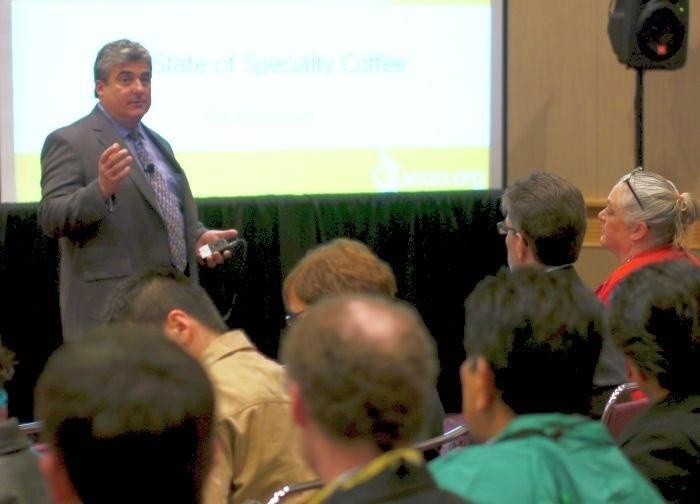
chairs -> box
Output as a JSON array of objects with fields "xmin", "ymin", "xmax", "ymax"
[
  {"xmin": 266, "ymin": 423, "xmax": 471, "ymax": 502},
  {"xmin": 602, "ymin": 381, "xmax": 655, "ymax": 437},
  {"xmin": 15, "ymin": 418, "xmax": 83, "ymax": 503}
]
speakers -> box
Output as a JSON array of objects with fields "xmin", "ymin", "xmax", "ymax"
[{"xmin": 607, "ymin": 0, "xmax": 690, "ymax": 71}]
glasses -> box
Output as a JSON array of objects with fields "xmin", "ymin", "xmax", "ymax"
[
  {"xmin": 622, "ymin": 166, "xmax": 652, "ymax": 208},
  {"xmin": 496, "ymin": 221, "xmax": 530, "ymax": 245},
  {"xmin": 284, "ymin": 308, "xmax": 307, "ymax": 326}
]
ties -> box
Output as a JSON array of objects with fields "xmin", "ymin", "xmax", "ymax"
[{"xmin": 129, "ymin": 131, "xmax": 187, "ymax": 274}]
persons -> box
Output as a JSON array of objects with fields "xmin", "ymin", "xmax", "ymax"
[
  {"xmin": 497, "ymin": 168, "xmax": 633, "ymax": 388},
  {"xmin": 592, "ymin": 166, "xmax": 700, "ymax": 402},
  {"xmin": 33, "ymin": 36, "xmax": 244, "ymax": 347},
  {"xmin": 2, "ymin": 239, "xmax": 700, "ymax": 504}
]
